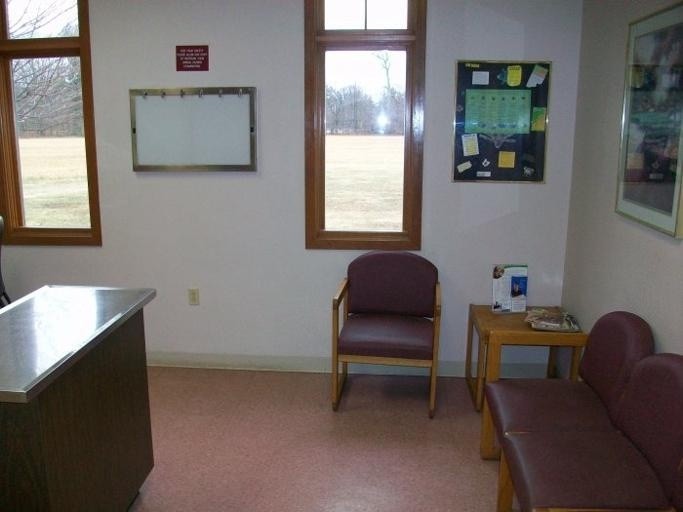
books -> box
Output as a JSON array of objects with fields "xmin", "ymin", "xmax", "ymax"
[{"xmin": 522, "ymin": 305, "xmax": 567, "ymax": 328}]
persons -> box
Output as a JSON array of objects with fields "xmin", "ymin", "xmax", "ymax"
[{"xmin": 511, "ymin": 281, "xmax": 522, "ymax": 296}]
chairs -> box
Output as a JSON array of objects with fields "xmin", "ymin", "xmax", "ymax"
[
  {"xmin": 478, "ymin": 311, "xmax": 657, "ymax": 510},
  {"xmin": 495, "ymin": 349, "xmax": 682, "ymax": 511},
  {"xmin": 326, "ymin": 250, "xmax": 443, "ymax": 419}
]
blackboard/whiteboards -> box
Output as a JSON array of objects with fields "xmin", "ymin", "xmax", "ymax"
[{"xmin": 128, "ymin": 87, "xmax": 257, "ymax": 172}]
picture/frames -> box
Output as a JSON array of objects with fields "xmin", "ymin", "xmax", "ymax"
[{"xmin": 613, "ymin": 0, "xmax": 682, "ymax": 240}]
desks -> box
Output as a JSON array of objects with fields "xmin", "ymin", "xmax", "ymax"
[{"xmin": 0, "ymin": 283, "xmax": 158, "ymax": 512}]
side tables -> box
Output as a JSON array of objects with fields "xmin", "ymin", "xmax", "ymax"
[{"xmin": 465, "ymin": 303, "xmax": 586, "ymax": 411}]
